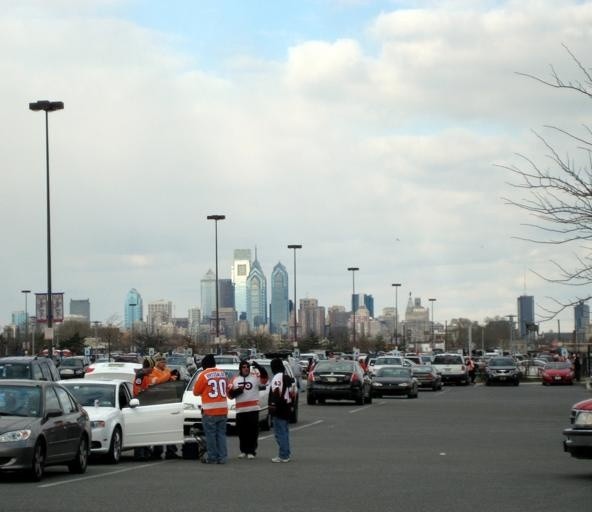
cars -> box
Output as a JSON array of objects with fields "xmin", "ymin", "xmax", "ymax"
[{"xmin": 563, "ymin": 398, "xmax": 591, "ymax": 460}]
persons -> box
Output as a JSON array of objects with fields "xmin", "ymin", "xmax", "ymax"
[
  {"xmin": 267, "ymin": 358, "xmax": 298, "ymax": 463},
  {"xmin": 193, "ymin": 354, "xmax": 230, "ymax": 465},
  {"xmin": 574, "ymin": 357, "xmax": 580, "ymax": 380},
  {"xmin": 133, "ymin": 357, "xmax": 154, "ymax": 462},
  {"xmin": 466, "ymin": 356, "xmax": 475, "ymax": 383},
  {"xmin": 227, "ymin": 361, "xmax": 269, "ymax": 459},
  {"xmin": 151, "ymin": 358, "xmax": 181, "ymax": 460}
]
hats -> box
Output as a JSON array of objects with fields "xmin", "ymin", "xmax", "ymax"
[{"xmin": 143, "ymin": 357, "xmax": 154, "ymax": 368}]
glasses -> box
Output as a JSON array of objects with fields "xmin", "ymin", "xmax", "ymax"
[{"xmin": 242, "ymin": 366, "xmax": 249, "ymax": 369}]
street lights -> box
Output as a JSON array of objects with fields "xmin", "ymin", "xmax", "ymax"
[
  {"xmin": 207, "ymin": 215, "xmax": 226, "ymax": 353},
  {"xmin": 22, "ymin": 288, "xmax": 31, "ymax": 352},
  {"xmin": 391, "ymin": 283, "xmax": 401, "ymax": 346},
  {"xmin": 503, "ymin": 313, "xmax": 518, "ymax": 352},
  {"xmin": 129, "ymin": 303, "xmax": 138, "ymax": 352},
  {"xmin": 428, "ymin": 298, "xmax": 436, "ymax": 351},
  {"xmin": 28, "ymin": 99, "xmax": 65, "ymax": 358},
  {"xmin": 287, "ymin": 244, "xmax": 302, "ymax": 357},
  {"xmin": 347, "ymin": 267, "xmax": 359, "ymax": 349},
  {"xmin": 91, "ymin": 319, "xmax": 103, "ymax": 361}
]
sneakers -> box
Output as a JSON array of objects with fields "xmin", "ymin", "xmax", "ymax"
[
  {"xmin": 133, "ymin": 454, "xmax": 182, "ymax": 462},
  {"xmin": 271, "ymin": 456, "xmax": 291, "ymax": 463},
  {"xmin": 200, "ymin": 456, "xmax": 224, "ymax": 465},
  {"xmin": 237, "ymin": 452, "xmax": 255, "ymax": 460}
]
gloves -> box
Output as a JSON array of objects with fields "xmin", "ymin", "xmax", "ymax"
[
  {"xmin": 230, "ymin": 388, "xmax": 243, "ymax": 398},
  {"xmin": 252, "ymin": 361, "xmax": 263, "ymax": 371}
]
